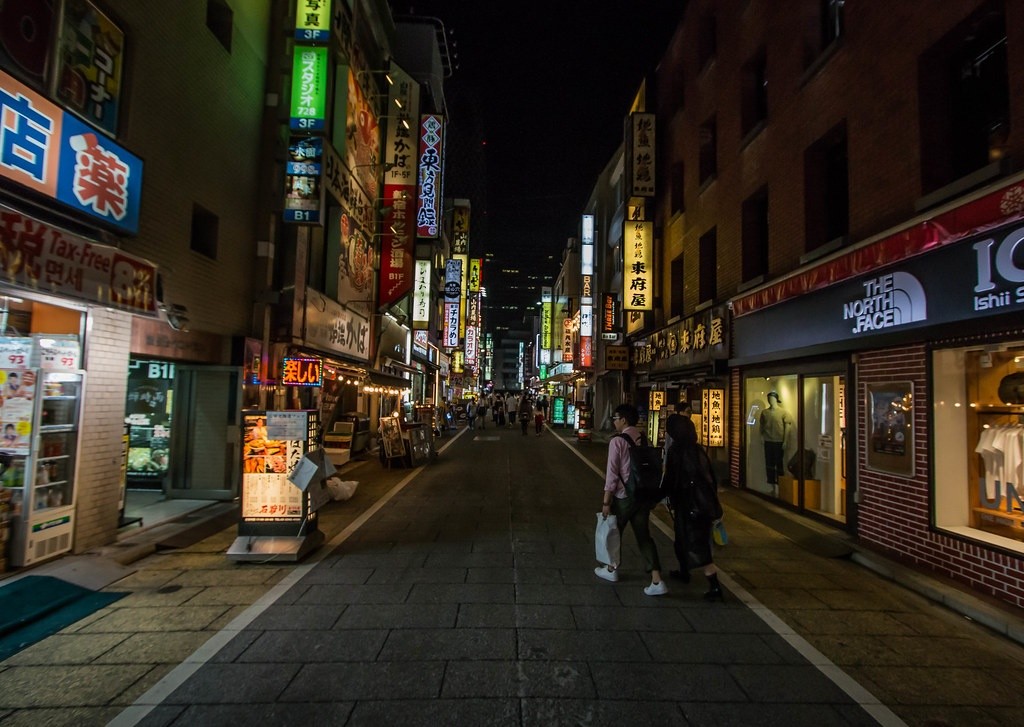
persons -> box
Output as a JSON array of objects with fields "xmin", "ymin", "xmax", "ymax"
[
  {"xmin": 3, "ymin": 374, "xmax": 26, "ymax": 401},
  {"xmin": 663, "ymin": 401, "xmax": 691, "ymax": 463},
  {"xmin": 595, "ymin": 402, "xmax": 669, "ymax": 596},
  {"xmin": 659, "ymin": 414, "xmax": 724, "ymax": 598},
  {"xmin": 0, "ymin": 422, "xmax": 18, "ymax": 449},
  {"xmin": 466, "ymin": 388, "xmax": 549, "ymax": 436},
  {"xmin": 758, "ymin": 392, "xmax": 792, "ymax": 496}
]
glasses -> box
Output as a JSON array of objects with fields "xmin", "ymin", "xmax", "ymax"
[
  {"xmin": 609, "ymin": 416, "xmax": 622, "ymax": 422},
  {"xmin": 685, "ymin": 410, "xmax": 693, "ymax": 414}
]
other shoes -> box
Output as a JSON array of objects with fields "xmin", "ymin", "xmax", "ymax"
[
  {"xmin": 536, "ymin": 432, "xmax": 542, "ymax": 436},
  {"xmin": 509, "ymin": 422, "xmax": 513, "ymax": 429}
]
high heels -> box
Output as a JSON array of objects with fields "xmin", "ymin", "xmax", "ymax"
[
  {"xmin": 670, "ymin": 568, "xmax": 694, "ymax": 593},
  {"xmin": 702, "ymin": 586, "xmax": 729, "ymax": 607}
]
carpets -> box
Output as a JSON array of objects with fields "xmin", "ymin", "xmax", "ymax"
[{"xmin": 0, "ymin": 574, "xmax": 133, "ymax": 663}]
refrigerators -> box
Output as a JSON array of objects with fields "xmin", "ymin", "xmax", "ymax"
[{"xmin": 11, "ymin": 368, "xmax": 88, "ymax": 568}]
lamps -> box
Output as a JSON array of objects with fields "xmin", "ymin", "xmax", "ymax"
[
  {"xmin": 367, "ymin": 313, "xmax": 406, "ymax": 327},
  {"xmin": 363, "ymin": 94, "xmax": 407, "ymax": 108},
  {"xmin": 375, "ymin": 116, "xmax": 415, "ymax": 129},
  {"xmin": 351, "ymin": 162, "xmax": 396, "ymax": 172},
  {"xmin": 976, "ymin": 351, "xmax": 993, "ymax": 369},
  {"xmin": 355, "ymin": 70, "xmax": 399, "ymax": 85},
  {"xmin": 343, "ymin": 300, "xmax": 389, "ymax": 314},
  {"xmin": 158, "ymin": 305, "xmax": 191, "ymax": 332},
  {"xmin": 355, "ymin": 190, "xmax": 412, "ymax": 245}
]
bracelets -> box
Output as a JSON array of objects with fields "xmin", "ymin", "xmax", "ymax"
[{"xmin": 602, "ymin": 503, "xmax": 609, "ymax": 506}]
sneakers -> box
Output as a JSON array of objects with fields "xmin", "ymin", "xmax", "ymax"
[
  {"xmin": 644, "ymin": 581, "xmax": 668, "ymax": 595},
  {"xmin": 593, "ymin": 565, "xmax": 618, "ymax": 582}
]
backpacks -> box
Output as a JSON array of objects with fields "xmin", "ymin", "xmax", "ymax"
[
  {"xmin": 788, "ymin": 447, "xmax": 817, "ymax": 480},
  {"xmin": 609, "ymin": 430, "xmax": 664, "ymax": 511},
  {"xmin": 671, "ymin": 444, "xmax": 723, "ymax": 523}
]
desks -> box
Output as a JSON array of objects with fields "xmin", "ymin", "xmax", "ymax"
[{"xmin": 778, "ymin": 476, "xmax": 822, "ymax": 510}]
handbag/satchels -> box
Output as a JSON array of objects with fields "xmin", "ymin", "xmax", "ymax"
[
  {"xmin": 478, "ymin": 406, "xmax": 486, "ymax": 417},
  {"xmin": 713, "ymin": 520, "xmax": 730, "ymax": 546},
  {"xmin": 594, "ymin": 512, "xmax": 620, "ymax": 569}
]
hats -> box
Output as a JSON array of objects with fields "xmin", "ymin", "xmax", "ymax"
[
  {"xmin": 544, "ymin": 395, "xmax": 547, "ymax": 398},
  {"xmin": 768, "ymin": 392, "xmax": 781, "ymax": 403}
]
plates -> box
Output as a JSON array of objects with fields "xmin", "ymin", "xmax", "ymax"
[{"xmin": 241, "ymin": 415, "xmax": 288, "ymax": 473}]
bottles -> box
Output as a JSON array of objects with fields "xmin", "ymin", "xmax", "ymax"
[{"xmin": 32, "ymin": 411, "xmax": 64, "ymax": 510}]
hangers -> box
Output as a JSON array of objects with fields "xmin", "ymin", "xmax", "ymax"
[{"xmin": 988, "ymin": 412, "xmax": 1024, "ymax": 429}]
